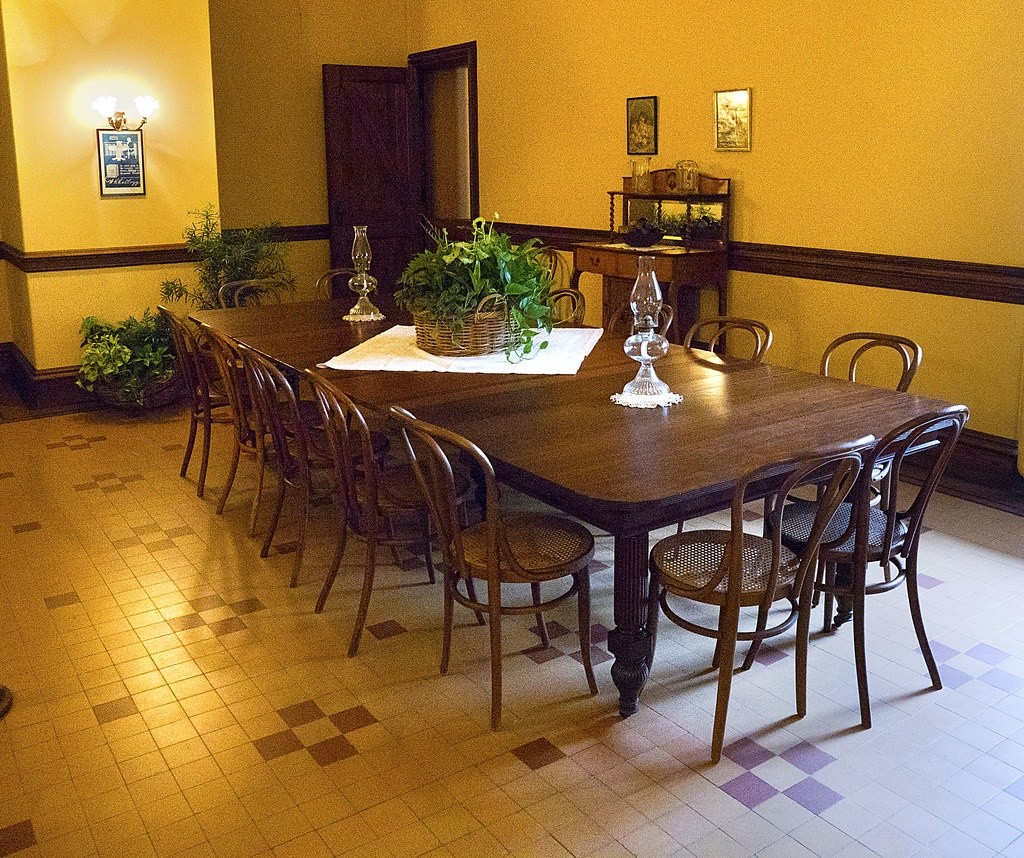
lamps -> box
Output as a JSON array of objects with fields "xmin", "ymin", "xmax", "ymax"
[{"xmin": 94, "ymin": 91, "xmax": 163, "ymax": 133}]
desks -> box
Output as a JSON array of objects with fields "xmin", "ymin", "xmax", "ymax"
[{"xmin": 186, "ymin": 301, "xmax": 972, "ymax": 721}]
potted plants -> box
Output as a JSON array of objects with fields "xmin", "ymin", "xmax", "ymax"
[
  {"xmin": 67, "ymin": 304, "xmax": 184, "ymax": 414},
  {"xmin": 392, "ymin": 211, "xmax": 564, "ymax": 368}
]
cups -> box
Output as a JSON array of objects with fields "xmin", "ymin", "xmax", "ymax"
[{"xmin": 632, "ymin": 157, "xmax": 650, "ymax": 191}]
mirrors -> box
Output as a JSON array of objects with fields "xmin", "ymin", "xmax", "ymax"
[{"xmin": 625, "ymin": 196, "xmax": 726, "ymax": 240}]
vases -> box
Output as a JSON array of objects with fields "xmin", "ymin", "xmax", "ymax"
[{"xmin": 340, "ymin": 225, "xmax": 387, "ymax": 322}]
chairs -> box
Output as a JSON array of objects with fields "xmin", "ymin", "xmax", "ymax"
[
  {"xmin": 235, "ymin": 339, "xmax": 411, "ymax": 587},
  {"xmin": 740, "ymin": 402, "xmax": 970, "ymax": 728},
  {"xmin": 299, "ymin": 367, "xmax": 488, "ymax": 659},
  {"xmin": 200, "ymin": 322, "xmax": 386, "ymax": 531},
  {"xmin": 607, "ymin": 297, "xmax": 674, "ymax": 339},
  {"xmin": 388, "ymin": 400, "xmax": 602, "ymax": 733},
  {"xmin": 148, "ymin": 303, "xmax": 332, "ymax": 497},
  {"xmin": 632, "ymin": 447, "xmax": 865, "ymax": 765},
  {"xmin": 314, "ymin": 267, "xmax": 379, "ymax": 301},
  {"xmin": 681, "ymin": 312, "xmax": 776, "ymax": 362},
  {"xmin": 547, "ymin": 283, "xmax": 587, "ymax": 327},
  {"xmin": 818, "ymin": 331, "xmax": 924, "ymax": 391},
  {"xmin": 217, "ymin": 276, "xmax": 295, "ymax": 309}
]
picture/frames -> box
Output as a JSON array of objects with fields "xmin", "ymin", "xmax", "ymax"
[
  {"xmin": 99, "ymin": 128, "xmax": 146, "ymax": 199},
  {"xmin": 624, "ymin": 94, "xmax": 660, "ymax": 156},
  {"xmin": 711, "ymin": 85, "xmax": 753, "ymax": 154}
]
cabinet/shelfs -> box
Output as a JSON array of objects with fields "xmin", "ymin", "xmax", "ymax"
[{"xmin": 568, "ymin": 167, "xmax": 732, "ymax": 355}]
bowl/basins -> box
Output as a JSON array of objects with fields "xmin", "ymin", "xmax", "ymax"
[{"xmin": 614, "ymin": 228, "xmax": 666, "ymax": 246}]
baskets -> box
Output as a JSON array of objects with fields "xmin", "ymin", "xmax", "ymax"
[{"xmin": 407, "ymin": 293, "xmax": 522, "ymax": 357}]
